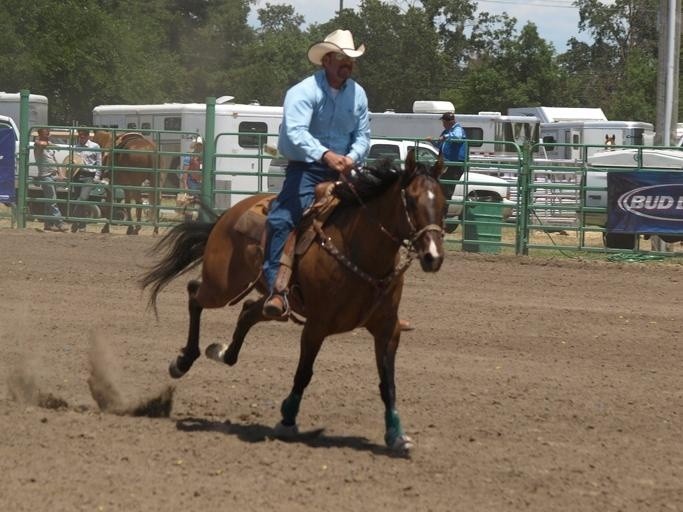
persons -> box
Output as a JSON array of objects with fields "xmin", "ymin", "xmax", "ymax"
[
  {"xmin": 33, "ymin": 128, "xmax": 68, "ymax": 232},
  {"xmin": 259, "ymin": 27, "xmax": 416, "ymax": 332},
  {"xmin": 70, "ymin": 124, "xmax": 103, "ymax": 233},
  {"xmin": 179, "ymin": 136, "xmax": 203, "ymax": 222},
  {"xmin": 422, "ymin": 111, "xmax": 469, "ymax": 219}
]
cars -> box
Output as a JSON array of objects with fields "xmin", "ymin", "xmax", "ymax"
[{"xmin": 25, "ymin": 126, "xmax": 97, "ymax": 143}]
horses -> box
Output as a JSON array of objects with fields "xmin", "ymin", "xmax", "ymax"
[
  {"xmin": 92, "ymin": 129, "xmax": 161, "ymax": 236},
  {"xmin": 604, "ymin": 133, "xmax": 617, "ymax": 152},
  {"xmin": 135, "ymin": 143, "xmax": 450, "ymax": 457}
]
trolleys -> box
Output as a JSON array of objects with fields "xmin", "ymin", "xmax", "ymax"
[{"xmin": 84, "ymin": 183, "xmax": 129, "ymax": 227}]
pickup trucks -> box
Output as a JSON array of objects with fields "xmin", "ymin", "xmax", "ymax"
[
  {"xmin": 0, "ymin": 115, "xmax": 94, "ymax": 215},
  {"xmin": 575, "ymin": 136, "xmax": 682, "ymax": 250},
  {"xmin": 267, "ymin": 136, "xmax": 514, "ymax": 234}
]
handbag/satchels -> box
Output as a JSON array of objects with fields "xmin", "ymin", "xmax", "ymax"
[{"xmin": 175, "ymin": 193, "xmax": 195, "ymax": 216}]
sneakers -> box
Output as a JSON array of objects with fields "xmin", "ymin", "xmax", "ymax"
[
  {"xmin": 59, "ymin": 222, "xmax": 69, "ymax": 231},
  {"xmin": 44, "ymin": 223, "xmax": 60, "ymax": 232}
]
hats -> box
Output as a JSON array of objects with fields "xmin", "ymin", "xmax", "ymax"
[
  {"xmin": 189, "ymin": 136, "xmax": 202, "ymax": 148},
  {"xmin": 439, "ymin": 112, "xmax": 455, "ymax": 121},
  {"xmin": 307, "ymin": 28, "xmax": 366, "ymax": 66},
  {"xmin": 61, "ymin": 155, "xmax": 84, "ymax": 179}
]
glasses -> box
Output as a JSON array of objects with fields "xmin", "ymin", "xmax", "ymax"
[{"xmin": 336, "ymin": 52, "xmax": 357, "ymax": 62}]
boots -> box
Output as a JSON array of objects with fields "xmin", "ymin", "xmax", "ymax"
[
  {"xmin": 397, "ymin": 316, "xmax": 415, "ymax": 332},
  {"xmin": 265, "ymin": 231, "xmax": 297, "ymax": 323}
]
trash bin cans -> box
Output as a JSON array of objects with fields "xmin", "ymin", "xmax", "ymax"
[{"xmin": 463, "ymin": 200, "xmax": 504, "ymax": 252}]
